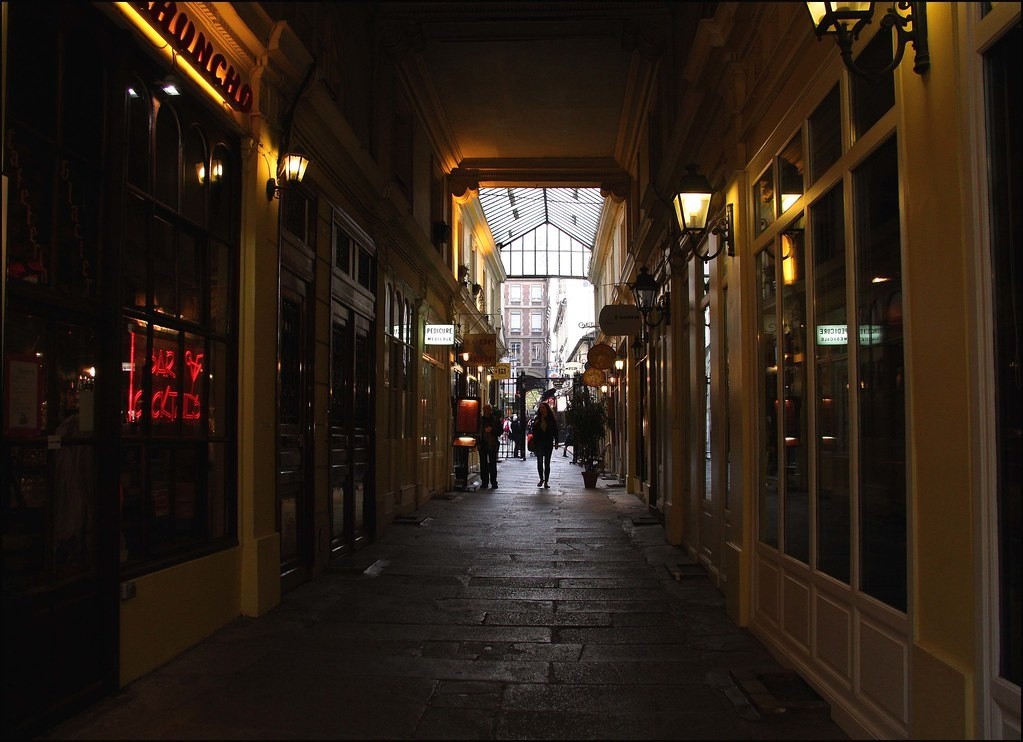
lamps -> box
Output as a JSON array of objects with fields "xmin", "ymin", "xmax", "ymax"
[
  {"xmin": 196, "ymin": 150, "xmax": 223, "ymax": 195},
  {"xmin": 629, "ymin": 267, "xmax": 670, "ymax": 328},
  {"xmin": 669, "ymin": 162, "xmax": 735, "ymax": 264},
  {"xmin": 162, "ymin": 47, "xmax": 183, "ymax": 95},
  {"xmin": 805, "ymin": 1, "xmax": 930, "ymax": 77},
  {"xmin": 631, "ymin": 335, "xmax": 647, "ymax": 362},
  {"xmin": 760, "ymin": 159, "xmax": 805, "ymax": 260},
  {"xmin": 267, "ymin": 150, "xmax": 310, "ymax": 201}
]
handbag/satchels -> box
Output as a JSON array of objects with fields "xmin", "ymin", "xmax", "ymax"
[{"xmin": 528, "ymin": 437, "xmax": 536, "ymax": 451}]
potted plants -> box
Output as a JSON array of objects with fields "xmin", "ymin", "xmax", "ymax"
[
  {"xmin": 565, "ymin": 387, "xmax": 616, "ymax": 488},
  {"xmin": 459, "ymin": 261, "xmax": 471, "ymax": 278},
  {"xmin": 472, "ymin": 283, "xmax": 485, "ymax": 317}
]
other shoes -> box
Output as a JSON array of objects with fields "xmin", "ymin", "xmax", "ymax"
[
  {"xmin": 480, "ymin": 482, "xmax": 488, "ymax": 488},
  {"xmin": 537, "ymin": 480, "xmax": 544, "ymax": 486},
  {"xmin": 492, "ymin": 484, "xmax": 498, "ymax": 489},
  {"xmin": 563, "ymin": 454, "xmax": 568, "ymax": 457},
  {"xmin": 544, "ymin": 484, "xmax": 550, "ymax": 488}
]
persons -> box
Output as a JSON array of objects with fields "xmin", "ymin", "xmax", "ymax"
[
  {"xmin": 476, "ymin": 403, "xmax": 504, "ymax": 489},
  {"xmin": 42, "ymin": 348, "xmax": 127, "ymax": 557},
  {"xmin": 509, "ymin": 403, "xmax": 573, "ymax": 488}
]
